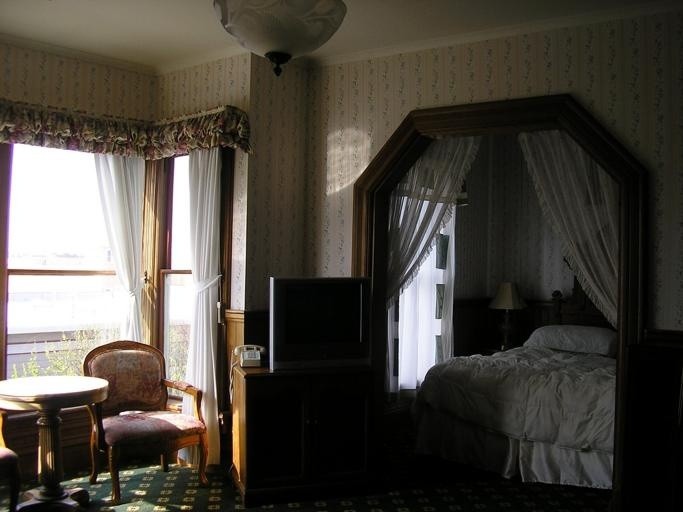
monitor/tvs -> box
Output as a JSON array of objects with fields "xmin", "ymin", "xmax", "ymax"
[{"xmin": 268, "ymin": 276, "xmax": 372, "ymax": 372}]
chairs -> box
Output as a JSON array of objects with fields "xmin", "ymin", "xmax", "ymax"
[{"xmin": 82, "ymin": 338, "xmax": 211, "ymax": 504}]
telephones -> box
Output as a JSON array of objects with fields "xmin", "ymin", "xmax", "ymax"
[{"xmin": 234, "ymin": 345, "xmax": 266, "ymax": 367}]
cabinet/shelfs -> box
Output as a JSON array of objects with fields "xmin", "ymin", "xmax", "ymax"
[{"xmin": 227, "ymin": 360, "xmax": 390, "ymax": 509}]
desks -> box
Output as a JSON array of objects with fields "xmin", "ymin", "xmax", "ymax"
[{"xmin": 0, "ymin": 374, "xmax": 110, "ymax": 508}]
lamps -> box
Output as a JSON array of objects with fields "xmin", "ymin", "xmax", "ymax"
[
  {"xmin": 489, "ymin": 281, "xmax": 525, "ymax": 347},
  {"xmin": 211, "ymin": 1, "xmax": 349, "ymax": 76}
]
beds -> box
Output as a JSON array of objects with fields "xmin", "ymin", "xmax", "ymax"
[{"xmin": 422, "ymin": 288, "xmax": 616, "ymax": 493}]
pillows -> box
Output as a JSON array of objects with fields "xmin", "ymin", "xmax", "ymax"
[{"xmin": 522, "ymin": 322, "xmax": 614, "ymax": 357}]
